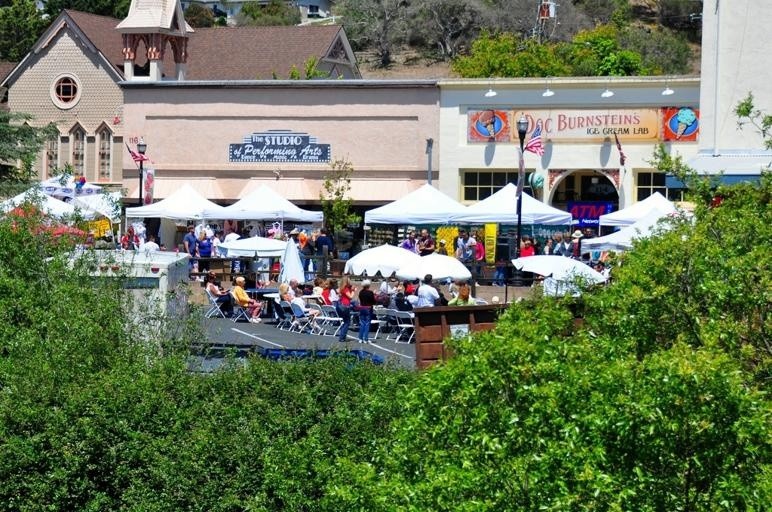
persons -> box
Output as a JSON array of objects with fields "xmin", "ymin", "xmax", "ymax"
[
  {"xmin": 492, "ymin": 246, "xmax": 505, "ymax": 287},
  {"xmin": 229, "ymin": 276, "xmax": 263, "ymax": 323},
  {"xmin": 183, "ymin": 224, "xmax": 240, "ymax": 282},
  {"xmin": 203, "ymin": 271, "xmax": 233, "ymax": 318},
  {"xmin": 399, "ymin": 229, "xmax": 485, "ymax": 287},
  {"xmin": 336, "ymin": 275, "xmax": 374, "ymax": 344},
  {"xmin": 519, "ymin": 227, "xmax": 595, "ymax": 264},
  {"xmin": 380, "ymin": 274, "xmax": 476, "ymax": 343},
  {"xmin": 250, "ymin": 272, "xmax": 340, "ymax": 334},
  {"xmin": 122, "ymin": 226, "xmax": 168, "ymax": 252},
  {"xmin": 268, "ymin": 226, "xmax": 332, "ymax": 279}
]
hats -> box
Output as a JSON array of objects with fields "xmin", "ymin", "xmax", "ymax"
[
  {"xmin": 572, "ymin": 230, "xmax": 583, "ymax": 238},
  {"xmin": 288, "ymin": 231, "xmax": 299, "ymax": 238},
  {"xmin": 268, "ymin": 229, "xmax": 277, "ymax": 234},
  {"xmin": 405, "ymin": 284, "xmax": 417, "ymax": 294},
  {"xmin": 438, "ymin": 239, "xmax": 446, "ymax": 245}
]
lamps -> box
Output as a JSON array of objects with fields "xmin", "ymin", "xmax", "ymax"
[
  {"xmin": 591, "ymin": 170, "xmax": 599, "ymax": 186},
  {"xmin": 601, "ymin": 89, "xmax": 613, "ymax": 99},
  {"xmin": 485, "ymin": 89, "xmax": 496, "ymax": 98},
  {"xmin": 542, "ymin": 89, "xmax": 554, "ymax": 96},
  {"xmin": 661, "ymin": 86, "xmax": 674, "ymax": 96}
]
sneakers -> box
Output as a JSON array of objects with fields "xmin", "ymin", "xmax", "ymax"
[
  {"xmin": 339, "ymin": 338, "xmax": 350, "ymax": 342},
  {"xmin": 248, "ymin": 317, "xmax": 262, "ymax": 324},
  {"xmin": 359, "ymin": 339, "xmax": 372, "ymax": 344}
]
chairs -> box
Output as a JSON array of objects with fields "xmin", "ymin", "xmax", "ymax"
[{"xmin": 202, "ymin": 287, "xmax": 414, "ymax": 346}]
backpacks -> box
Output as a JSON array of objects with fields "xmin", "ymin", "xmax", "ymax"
[{"xmin": 397, "ymin": 295, "xmax": 413, "ymax": 311}]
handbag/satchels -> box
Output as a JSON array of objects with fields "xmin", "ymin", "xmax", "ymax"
[{"xmin": 336, "ymin": 300, "xmax": 350, "ymax": 318}]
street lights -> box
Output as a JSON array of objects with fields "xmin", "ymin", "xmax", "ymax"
[
  {"xmin": 134, "ymin": 138, "xmax": 147, "ymax": 203},
  {"xmin": 503, "ymin": 113, "xmax": 529, "ymax": 286}
]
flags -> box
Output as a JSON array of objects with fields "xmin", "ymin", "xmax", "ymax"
[
  {"xmin": 525, "ymin": 123, "xmax": 544, "ymax": 157},
  {"xmin": 127, "ymin": 142, "xmax": 151, "ymax": 161}
]
